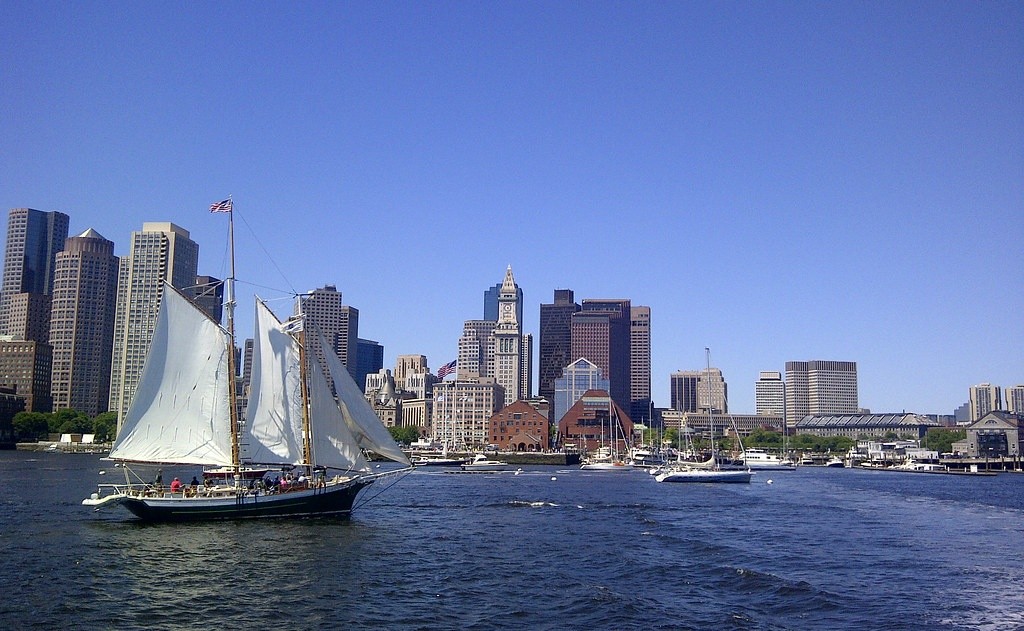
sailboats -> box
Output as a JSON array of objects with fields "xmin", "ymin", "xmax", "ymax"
[{"xmin": 80, "ymin": 195, "xmax": 418, "ymax": 522}]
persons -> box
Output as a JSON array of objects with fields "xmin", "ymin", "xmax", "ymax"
[
  {"xmin": 189, "ymin": 477, "xmax": 199, "ymax": 486},
  {"xmin": 169, "ymin": 477, "xmax": 183, "ymax": 493},
  {"xmin": 202, "ymin": 477, "xmax": 212, "ymax": 488},
  {"xmin": 255, "ymin": 473, "xmax": 323, "ymax": 495},
  {"xmin": 154, "ymin": 470, "xmax": 163, "ymax": 496}
]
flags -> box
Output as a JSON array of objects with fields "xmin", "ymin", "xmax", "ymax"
[
  {"xmin": 209, "ymin": 199, "xmax": 231, "ymax": 214},
  {"xmin": 436, "ymin": 361, "xmax": 455, "ymax": 379}
]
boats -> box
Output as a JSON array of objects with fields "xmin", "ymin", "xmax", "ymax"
[
  {"xmin": 372, "ymin": 345, "xmax": 1024, "ymax": 484},
  {"xmin": 42, "ymin": 438, "xmax": 114, "ymax": 455}
]
